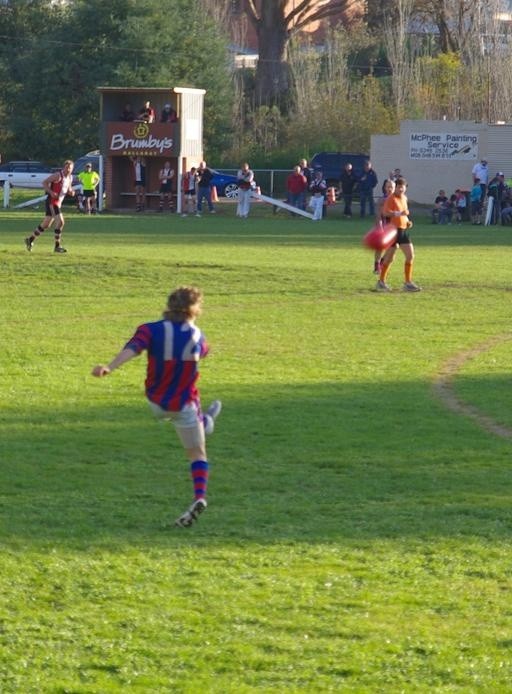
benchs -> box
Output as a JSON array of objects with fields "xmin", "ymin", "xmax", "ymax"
[{"xmin": 410, "ymin": 208, "xmax": 462, "ymax": 225}]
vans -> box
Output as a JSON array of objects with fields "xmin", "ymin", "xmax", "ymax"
[{"xmin": 65, "ymin": 150, "xmax": 101, "ymax": 201}]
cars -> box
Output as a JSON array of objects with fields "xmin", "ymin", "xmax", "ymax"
[
  {"xmin": 1, "ymin": 160, "xmax": 59, "ymax": 187},
  {"xmin": 205, "ymin": 166, "xmax": 256, "ymax": 199}
]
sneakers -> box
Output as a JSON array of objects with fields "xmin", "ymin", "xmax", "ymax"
[
  {"xmin": 24, "ymin": 237, "xmax": 34, "ymax": 253},
  {"xmin": 54, "ymin": 247, "xmax": 67, "ymax": 254},
  {"xmin": 204, "ymin": 400, "xmax": 221, "ymax": 434},
  {"xmin": 172, "ymin": 497, "xmax": 207, "ymax": 529},
  {"xmin": 375, "ymin": 280, "xmax": 393, "ymax": 292},
  {"xmin": 403, "ymin": 282, "xmax": 421, "ymax": 293}
]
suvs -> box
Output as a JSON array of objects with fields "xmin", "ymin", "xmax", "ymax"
[{"xmin": 303, "ymin": 150, "xmax": 370, "ymax": 200}]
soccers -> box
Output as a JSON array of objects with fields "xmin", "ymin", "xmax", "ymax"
[{"xmin": 367, "ymin": 222, "xmax": 400, "ymax": 248}]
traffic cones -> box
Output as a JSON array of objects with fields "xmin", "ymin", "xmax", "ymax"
[
  {"xmin": 209, "ymin": 184, "xmax": 220, "ymax": 202},
  {"xmin": 325, "ymin": 184, "xmax": 337, "ymax": 205},
  {"xmin": 253, "ymin": 186, "xmax": 263, "ymax": 202}
]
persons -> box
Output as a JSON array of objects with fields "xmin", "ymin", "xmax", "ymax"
[
  {"xmin": 24, "ymin": 159, "xmax": 75, "ymax": 253},
  {"xmin": 196, "ymin": 161, "xmax": 215, "ymax": 214},
  {"xmin": 180, "ymin": 166, "xmax": 200, "ymax": 216},
  {"xmin": 137, "ymin": 100, "xmax": 155, "ymax": 124},
  {"xmin": 234, "ymin": 163, "xmax": 254, "ymax": 220},
  {"xmin": 372, "ymin": 179, "xmax": 396, "ymax": 274},
  {"xmin": 130, "ymin": 156, "xmax": 147, "ymax": 212},
  {"xmin": 287, "ymin": 156, "xmax": 404, "ymax": 219},
  {"xmin": 120, "ymin": 102, "xmax": 138, "ymax": 121},
  {"xmin": 430, "ymin": 156, "xmax": 511, "ymax": 226},
  {"xmin": 90, "ymin": 285, "xmax": 222, "ymax": 526},
  {"xmin": 157, "ymin": 160, "xmax": 175, "ymax": 212},
  {"xmin": 78, "ymin": 162, "xmax": 100, "ymax": 215},
  {"xmin": 376, "ymin": 176, "xmax": 422, "ymax": 291},
  {"xmin": 159, "ymin": 103, "xmax": 176, "ymax": 122}
]
